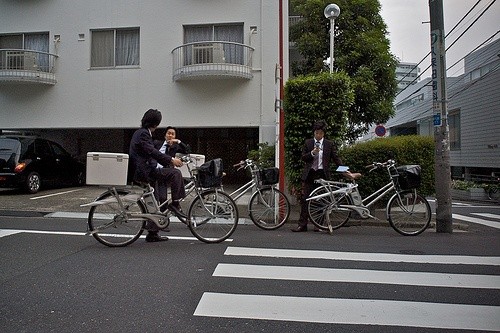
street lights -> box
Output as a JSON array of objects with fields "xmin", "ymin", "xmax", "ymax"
[{"xmin": 324, "ymin": 3, "xmax": 341, "ymax": 74}]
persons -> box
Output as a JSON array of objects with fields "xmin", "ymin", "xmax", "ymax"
[
  {"xmin": 153, "ymin": 127, "xmax": 189, "ymax": 232},
  {"xmin": 127, "ymin": 110, "xmax": 187, "ymax": 242},
  {"xmin": 290, "ymin": 122, "xmax": 351, "ymax": 232}
]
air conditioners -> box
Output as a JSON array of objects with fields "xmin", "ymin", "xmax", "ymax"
[
  {"xmin": 5, "ymin": 50, "xmax": 38, "ymax": 71},
  {"xmin": 191, "ymin": 44, "xmax": 224, "ymax": 63}
]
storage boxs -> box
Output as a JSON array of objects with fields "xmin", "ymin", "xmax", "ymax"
[
  {"xmin": 174, "ymin": 154, "xmax": 205, "ymax": 177},
  {"xmin": 85, "ymin": 151, "xmax": 129, "ymax": 187}
]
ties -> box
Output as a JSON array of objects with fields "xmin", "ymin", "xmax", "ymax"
[
  {"xmin": 165, "ymin": 144, "xmax": 168, "ymax": 155},
  {"xmin": 313, "ymin": 142, "xmax": 318, "ymax": 171}
]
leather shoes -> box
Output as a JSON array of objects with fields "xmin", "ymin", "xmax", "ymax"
[
  {"xmin": 314, "ymin": 226, "xmax": 324, "ymax": 231},
  {"xmin": 167, "ymin": 204, "xmax": 188, "ymax": 218},
  {"xmin": 146, "ymin": 235, "xmax": 167, "ymax": 241},
  {"xmin": 290, "ymin": 226, "xmax": 307, "ymax": 232}
]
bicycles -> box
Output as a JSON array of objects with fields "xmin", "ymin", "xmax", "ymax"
[
  {"xmin": 487, "ymin": 178, "xmax": 500, "ymax": 200},
  {"xmin": 305, "ymin": 159, "xmax": 432, "ymax": 236},
  {"xmin": 171, "ymin": 159, "xmax": 290, "ymax": 230},
  {"xmin": 85, "ymin": 155, "xmax": 240, "ymax": 247}
]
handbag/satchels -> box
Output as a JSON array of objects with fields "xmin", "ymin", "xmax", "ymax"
[
  {"xmin": 397, "ymin": 166, "xmax": 421, "ymax": 188},
  {"xmin": 198, "ymin": 158, "xmax": 222, "ymax": 187},
  {"xmin": 260, "ymin": 168, "xmax": 279, "ymax": 185}
]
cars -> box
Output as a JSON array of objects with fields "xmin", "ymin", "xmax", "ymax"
[{"xmin": 0, "ymin": 135, "xmax": 86, "ymax": 194}]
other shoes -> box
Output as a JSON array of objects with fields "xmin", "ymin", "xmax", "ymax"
[{"xmin": 162, "ymin": 228, "xmax": 169, "ymax": 231}]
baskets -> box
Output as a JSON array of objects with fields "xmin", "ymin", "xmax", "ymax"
[
  {"xmin": 258, "ymin": 168, "xmax": 279, "ymax": 186},
  {"xmin": 192, "ymin": 157, "xmax": 222, "ymax": 187},
  {"xmin": 393, "ymin": 165, "xmax": 421, "ymax": 189}
]
